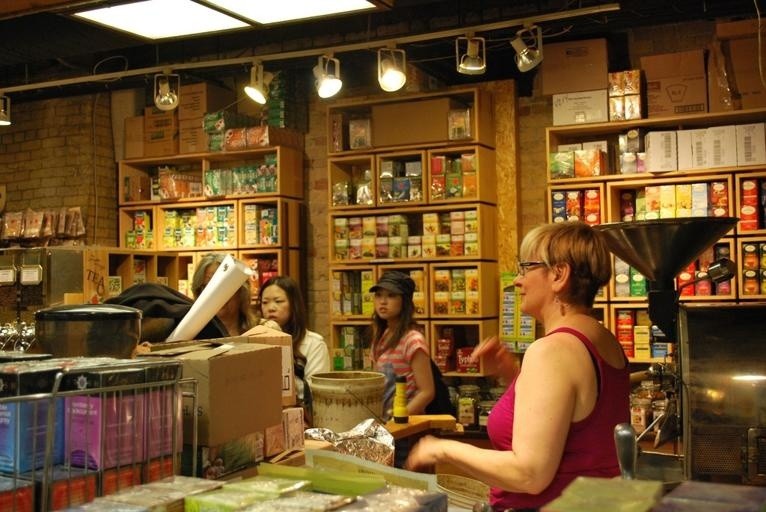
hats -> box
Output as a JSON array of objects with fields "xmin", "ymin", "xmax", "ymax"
[{"xmin": 369, "ymin": 270, "xmax": 415, "ymax": 295}]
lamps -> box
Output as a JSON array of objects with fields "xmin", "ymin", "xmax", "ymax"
[{"xmin": 0, "ymin": 0, "xmax": 623, "ymax": 126}]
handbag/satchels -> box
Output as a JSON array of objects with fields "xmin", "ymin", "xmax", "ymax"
[{"xmin": 424, "ymin": 357, "xmax": 456, "ymax": 417}]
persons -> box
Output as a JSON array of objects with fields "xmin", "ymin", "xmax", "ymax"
[
  {"xmin": 367, "ymin": 270, "xmax": 458, "ymax": 416},
  {"xmin": 191, "ymin": 253, "xmax": 284, "ymax": 337},
  {"xmin": 257, "ymin": 275, "xmax": 331, "ymax": 429},
  {"xmin": 402, "ymin": 220, "xmax": 635, "ymax": 512}
]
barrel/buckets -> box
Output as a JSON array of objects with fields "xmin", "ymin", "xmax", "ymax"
[{"xmin": 309, "ymin": 371, "xmax": 390, "ymax": 438}]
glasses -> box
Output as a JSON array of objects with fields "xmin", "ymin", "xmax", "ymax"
[{"xmin": 517, "ymin": 261, "xmax": 545, "ymax": 275}]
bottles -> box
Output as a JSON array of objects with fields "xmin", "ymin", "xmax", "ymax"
[
  {"xmin": 392, "ymin": 377, "xmax": 412, "ymax": 424},
  {"xmin": 455, "ymin": 383, "xmax": 506, "ymax": 438},
  {"xmin": 633, "ymin": 379, "xmax": 673, "ymax": 436}
]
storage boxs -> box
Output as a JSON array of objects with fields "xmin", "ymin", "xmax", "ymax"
[
  {"xmin": 370, "ymin": 97, "xmax": 450, "ymax": 146},
  {"xmin": 135, "ymin": 325, "xmax": 296, "ymax": 447},
  {"xmin": 540, "ymin": 18, "xmax": 766, "ymax": 126},
  {"xmin": 126, "ymin": 77, "xmax": 301, "ymax": 156}
]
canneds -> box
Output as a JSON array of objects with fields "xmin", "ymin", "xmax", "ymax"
[{"xmin": 448, "ymin": 384, "xmax": 508, "ymax": 434}]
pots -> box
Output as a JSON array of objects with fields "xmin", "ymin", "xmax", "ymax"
[{"xmin": 30, "ymin": 299, "xmax": 146, "ymax": 359}]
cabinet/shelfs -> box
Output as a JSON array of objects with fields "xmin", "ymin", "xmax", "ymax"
[
  {"xmin": 323, "ymin": 87, "xmax": 498, "ymax": 378},
  {"xmin": 544, "ymin": 109, "xmax": 766, "ymax": 458},
  {"xmin": 85, "ymin": 149, "xmax": 305, "ymax": 307}
]
